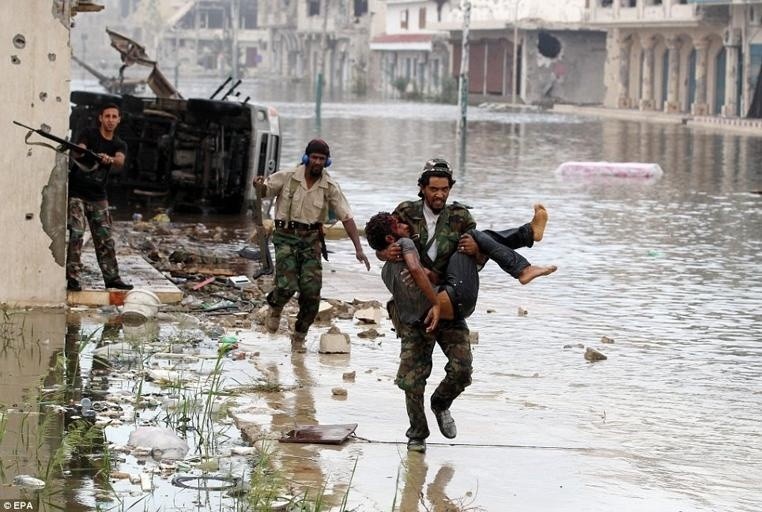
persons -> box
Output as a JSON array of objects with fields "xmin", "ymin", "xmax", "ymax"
[
  {"xmin": 252, "ymin": 139, "xmax": 371, "ymax": 352},
  {"xmin": 375, "ymin": 158, "xmax": 490, "ymax": 453},
  {"xmin": 366, "ymin": 202, "xmax": 558, "ymax": 333},
  {"xmin": 64, "ymin": 103, "xmax": 134, "ymax": 292}
]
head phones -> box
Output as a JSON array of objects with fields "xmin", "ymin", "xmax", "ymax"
[{"xmin": 302, "ymin": 138, "xmax": 332, "ymax": 169}]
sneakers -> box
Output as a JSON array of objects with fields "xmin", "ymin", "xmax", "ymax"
[
  {"xmin": 106, "ymin": 279, "xmax": 134, "ymax": 290},
  {"xmin": 68, "ymin": 279, "xmax": 82, "ymax": 291},
  {"xmin": 266, "ymin": 305, "xmax": 283, "ymax": 334},
  {"xmin": 407, "ymin": 437, "xmax": 425, "ymax": 451},
  {"xmin": 431, "ymin": 407, "xmax": 457, "ymax": 439},
  {"xmin": 292, "ymin": 337, "xmax": 308, "ymax": 354}
]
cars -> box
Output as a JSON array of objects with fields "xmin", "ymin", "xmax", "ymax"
[{"xmin": 68, "ymin": 90, "xmax": 282, "ymax": 218}]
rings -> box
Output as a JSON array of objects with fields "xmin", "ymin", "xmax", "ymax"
[
  {"xmin": 460, "ymin": 245, "xmax": 465, "ymax": 251},
  {"xmin": 396, "ymin": 254, "xmax": 401, "ymax": 260}
]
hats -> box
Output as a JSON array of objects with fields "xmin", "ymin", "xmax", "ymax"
[
  {"xmin": 420, "ymin": 158, "xmax": 453, "ymax": 178},
  {"xmin": 307, "ymin": 139, "xmax": 330, "ymax": 157}
]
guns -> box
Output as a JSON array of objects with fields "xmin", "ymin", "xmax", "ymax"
[
  {"xmin": 13, "ymin": 121, "xmax": 113, "ymax": 170},
  {"xmin": 239, "ymin": 181, "xmax": 274, "ymax": 280}
]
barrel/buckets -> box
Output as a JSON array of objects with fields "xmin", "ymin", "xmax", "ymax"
[{"xmin": 120, "ymin": 288, "xmax": 160, "ymax": 327}]
